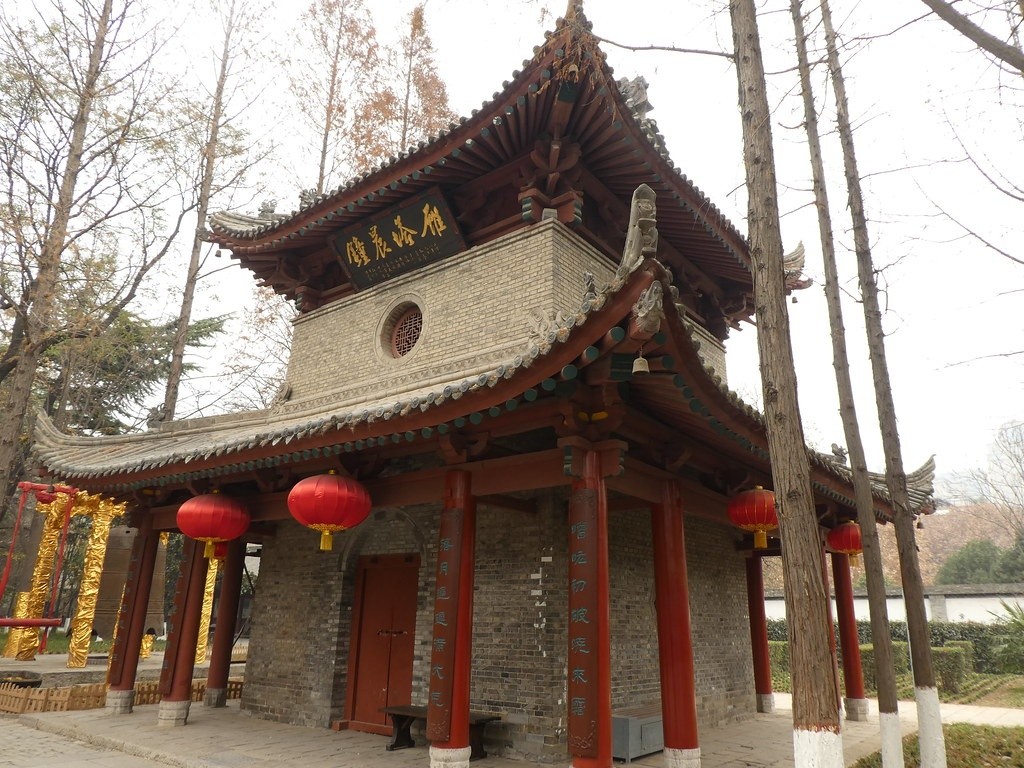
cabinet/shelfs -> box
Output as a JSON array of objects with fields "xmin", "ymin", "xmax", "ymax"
[{"xmin": 613, "ymin": 708, "xmax": 665, "ymax": 764}]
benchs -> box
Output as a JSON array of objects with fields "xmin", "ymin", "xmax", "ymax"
[{"xmin": 380, "ymin": 705, "xmax": 501, "ymax": 762}]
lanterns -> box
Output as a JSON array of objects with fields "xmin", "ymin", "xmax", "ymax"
[
  {"xmin": 288, "ymin": 470, "xmax": 371, "ymax": 549},
  {"xmin": 828, "ymin": 519, "xmax": 864, "ymax": 566},
  {"xmin": 176, "ymin": 493, "xmax": 248, "ymax": 558},
  {"xmin": 728, "ymin": 485, "xmax": 779, "ymax": 547}
]
